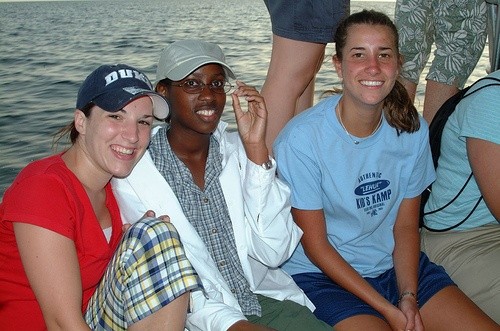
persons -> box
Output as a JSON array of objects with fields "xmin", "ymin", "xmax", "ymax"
[
  {"xmin": 0, "ymin": 63, "xmax": 209, "ymax": 331},
  {"xmin": 269, "ymin": 10, "xmax": 500, "ymax": 331},
  {"xmin": 418, "ymin": 68, "xmax": 500, "ymax": 330},
  {"xmin": 260, "ymin": 0, "xmax": 351, "ymax": 159},
  {"xmin": 108, "ymin": 37, "xmax": 339, "ymax": 331},
  {"xmin": 392, "ymin": 0, "xmax": 491, "ymax": 126}
]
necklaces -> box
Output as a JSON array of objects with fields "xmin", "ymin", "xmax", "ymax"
[{"xmin": 339, "ymin": 96, "xmax": 384, "ymax": 144}]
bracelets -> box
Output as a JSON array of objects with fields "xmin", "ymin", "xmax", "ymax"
[
  {"xmin": 258, "ymin": 161, "xmax": 273, "ymax": 169},
  {"xmin": 397, "ymin": 292, "xmax": 419, "ymax": 306}
]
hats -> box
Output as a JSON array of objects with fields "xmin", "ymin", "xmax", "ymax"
[
  {"xmin": 153, "ymin": 38, "xmax": 236, "ymax": 83},
  {"xmin": 74, "ymin": 64, "xmax": 172, "ymax": 120}
]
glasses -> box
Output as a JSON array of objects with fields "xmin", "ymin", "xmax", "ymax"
[{"xmin": 161, "ymin": 78, "xmax": 235, "ymax": 94}]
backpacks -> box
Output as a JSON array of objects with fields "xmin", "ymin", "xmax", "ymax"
[{"xmin": 417, "ymin": 76, "xmax": 499, "ymax": 232}]
